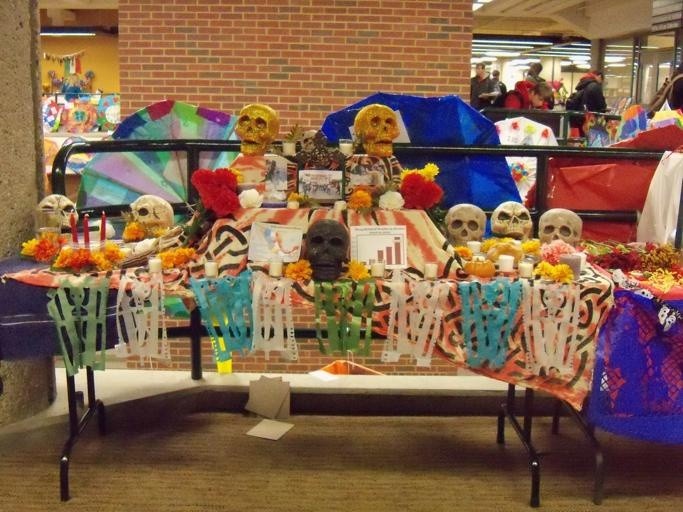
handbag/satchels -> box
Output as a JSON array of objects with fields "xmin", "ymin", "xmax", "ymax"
[
  {"xmin": 566, "ymin": 90, "xmax": 585, "ymax": 128},
  {"xmin": 492, "ymin": 90, "xmax": 523, "ymax": 108}
]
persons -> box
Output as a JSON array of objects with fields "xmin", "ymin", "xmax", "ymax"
[
  {"xmin": 646, "ymin": 61, "xmax": 683, "ymax": 119},
  {"xmin": 471, "ymin": 62, "xmax": 503, "ymax": 111},
  {"xmin": 575, "ymin": 70, "xmax": 607, "ymax": 137},
  {"xmin": 525, "ymin": 63, "xmax": 554, "ymax": 109},
  {"xmin": 490, "ymin": 69, "xmax": 507, "ymax": 105},
  {"xmin": 493, "ymin": 81, "xmax": 553, "ymax": 110}
]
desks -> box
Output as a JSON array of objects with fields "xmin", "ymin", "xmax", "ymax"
[{"xmin": 0, "ymin": 263, "xmax": 683, "ymax": 510}]
